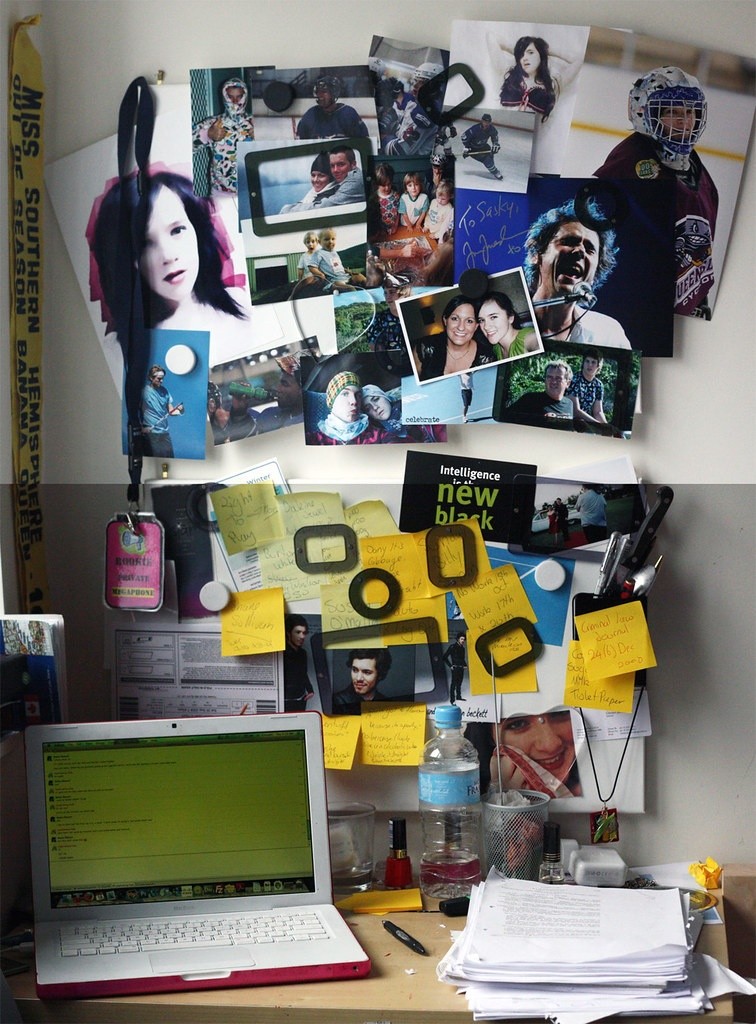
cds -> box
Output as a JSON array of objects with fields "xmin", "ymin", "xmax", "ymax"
[{"xmin": 683, "ymin": 889, "xmax": 717, "ymax": 913}]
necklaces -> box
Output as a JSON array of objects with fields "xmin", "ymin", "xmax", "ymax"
[
  {"xmin": 446, "ymin": 341, "xmax": 472, "ymax": 360},
  {"xmin": 580, "ymin": 686, "xmax": 644, "ymax": 844}
]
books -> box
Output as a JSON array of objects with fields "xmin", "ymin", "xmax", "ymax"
[{"xmin": 0, "ymin": 614, "xmax": 66, "ymax": 727}]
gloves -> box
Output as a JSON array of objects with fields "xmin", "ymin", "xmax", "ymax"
[
  {"xmin": 491, "ymin": 144, "xmax": 500, "ymax": 154},
  {"xmin": 463, "ymin": 148, "xmax": 471, "ymax": 159}
]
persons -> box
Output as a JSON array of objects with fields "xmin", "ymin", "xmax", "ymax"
[
  {"xmin": 458, "ymin": 370, "xmax": 477, "ymax": 423},
  {"xmin": 591, "ymin": 65, "xmax": 719, "ymax": 321},
  {"xmin": 283, "ymin": 615, "xmax": 314, "ymax": 713},
  {"xmin": 564, "ymin": 349, "xmax": 607, "ymax": 424},
  {"xmin": 547, "ymin": 497, "xmax": 571, "ymax": 544},
  {"xmin": 574, "ymin": 484, "xmax": 608, "ymax": 542},
  {"xmin": 461, "ymin": 114, "xmax": 503, "ymax": 180},
  {"xmin": 500, "ymin": 36, "xmax": 560, "ymax": 122},
  {"xmin": 138, "ymin": 365, "xmax": 185, "ymax": 458},
  {"xmin": 522, "ymin": 196, "xmax": 632, "ymax": 350},
  {"xmin": 490, "ymin": 711, "xmax": 583, "ymax": 799},
  {"xmin": 506, "ymin": 359, "xmax": 573, "ymax": 419},
  {"xmin": 207, "ymin": 350, "xmax": 318, "ymax": 445},
  {"xmin": 443, "ymin": 633, "xmax": 468, "ymax": 706},
  {"xmin": 332, "ymin": 648, "xmax": 391, "ymax": 704},
  {"xmin": 412, "ymin": 291, "xmax": 539, "ymax": 383},
  {"xmin": 306, "ymin": 370, "xmax": 400, "ymax": 445},
  {"xmin": 361, "ymin": 384, "xmax": 438, "ymax": 443},
  {"xmin": 92, "ymin": 33, "xmax": 455, "ymax": 366}
]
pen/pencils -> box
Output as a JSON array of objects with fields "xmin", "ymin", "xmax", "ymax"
[
  {"xmin": 593, "ymin": 529, "xmax": 664, "ymax": 601},
  {"xmin": 382, "ymin": 918, "xmax": 426, "ymax": 956}
]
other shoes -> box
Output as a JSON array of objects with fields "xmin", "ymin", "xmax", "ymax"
[{"xmin": 463, "ymin": 416, "xmax": 468, "ymax": 423}]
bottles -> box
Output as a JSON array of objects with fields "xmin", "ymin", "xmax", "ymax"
[{"xmin": 418, "ymin": 705, "xmax": 482, "ymax": 898}]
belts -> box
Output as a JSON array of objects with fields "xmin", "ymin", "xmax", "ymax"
[{"xmin": 285, "ymin": 693, "xmax": 306, "ymax": 701}]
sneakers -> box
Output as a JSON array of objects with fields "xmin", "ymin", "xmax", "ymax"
[{"xmin": 493, "ymin": 171, "xmax": 503, "ymax": 182}]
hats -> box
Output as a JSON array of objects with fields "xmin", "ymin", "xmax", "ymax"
[
  {"xmin": 311, "ymin": 151, "xmax": 335, "ymax": 181},
  {"xmin": 208, "ymin": 382, "xmax": 222, "ymax": 409},
  {"xmin": 326, "ymin": 372, "xmax": 363, "ymax": 410},
  {"xmin": 274, "ymin": 348, "xmax": 317, "ymax": 387},
  {"xmin": 482, "ymin": 114, "xmax": 492, "ymax": 122}
]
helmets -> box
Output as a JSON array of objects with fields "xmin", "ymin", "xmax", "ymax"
[
  {"xmin": 430, "ymin": 153, "xmax": 449, "ymax": 167},
  {"xmin": 628, "ymin": 65, "xmax": 707, "ymax": 170},
  {"xmin": 369, "ymin": 57, "xmax": 386, "ymax": 78},
  {"xmin": 314, "ymin": 75, "xmax": 342, "ymax": 98},
  {"xmin": 376, "ymin": 77, "xmax": 397, "ymax": 106},
  {"xmin": 414, "ymin": 63, "xmax": 444, "ymax": 83}
]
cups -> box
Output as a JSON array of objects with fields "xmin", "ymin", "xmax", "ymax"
[{"xmin": 326, "ymin": 801, "xmax": 377, "ymax": 893}]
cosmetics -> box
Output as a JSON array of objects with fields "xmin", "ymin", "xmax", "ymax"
[{"xmin": 385, "ymin": 817, "xmax": 413, "ymax": 886}]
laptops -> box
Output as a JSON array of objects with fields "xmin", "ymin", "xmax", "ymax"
[{"xmin": 24, "ymin": 711, "xmax": 371, "ymax": 999}]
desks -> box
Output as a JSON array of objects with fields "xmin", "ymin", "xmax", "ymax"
[{"xmin": 0, "ymin": 878, "xmax": 735, "ymax": 1024}]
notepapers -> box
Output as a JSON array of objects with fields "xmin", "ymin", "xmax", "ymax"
[{"xmin": 350, "ymin": 889, "xmax": 423, "ymax": 913}]
